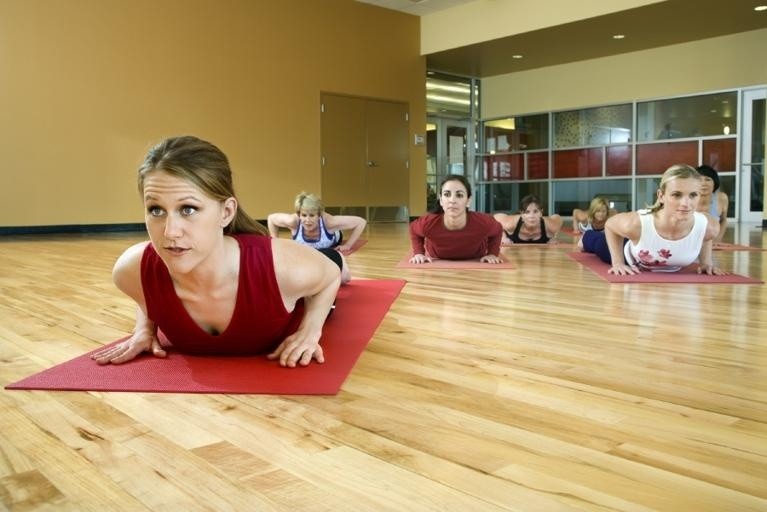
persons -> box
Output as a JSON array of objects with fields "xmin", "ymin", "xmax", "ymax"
[
  {"xmin": 571, "ymin": 164, "xmax": 731, "ymax": 276},
  {"xmin": 267, "ymin": 191, "xmax": 367, "ymax": 252},
  {"xmin": 89, "ymin": 135, "xmax": 351, "ymax": 369},
  {"xmin": 492, "ymin": 195, "xmax": 563, "ymax": 246},
  {"xmin": 408, "ymin": 175, "xmax": 504, "ymax": 264}
]
